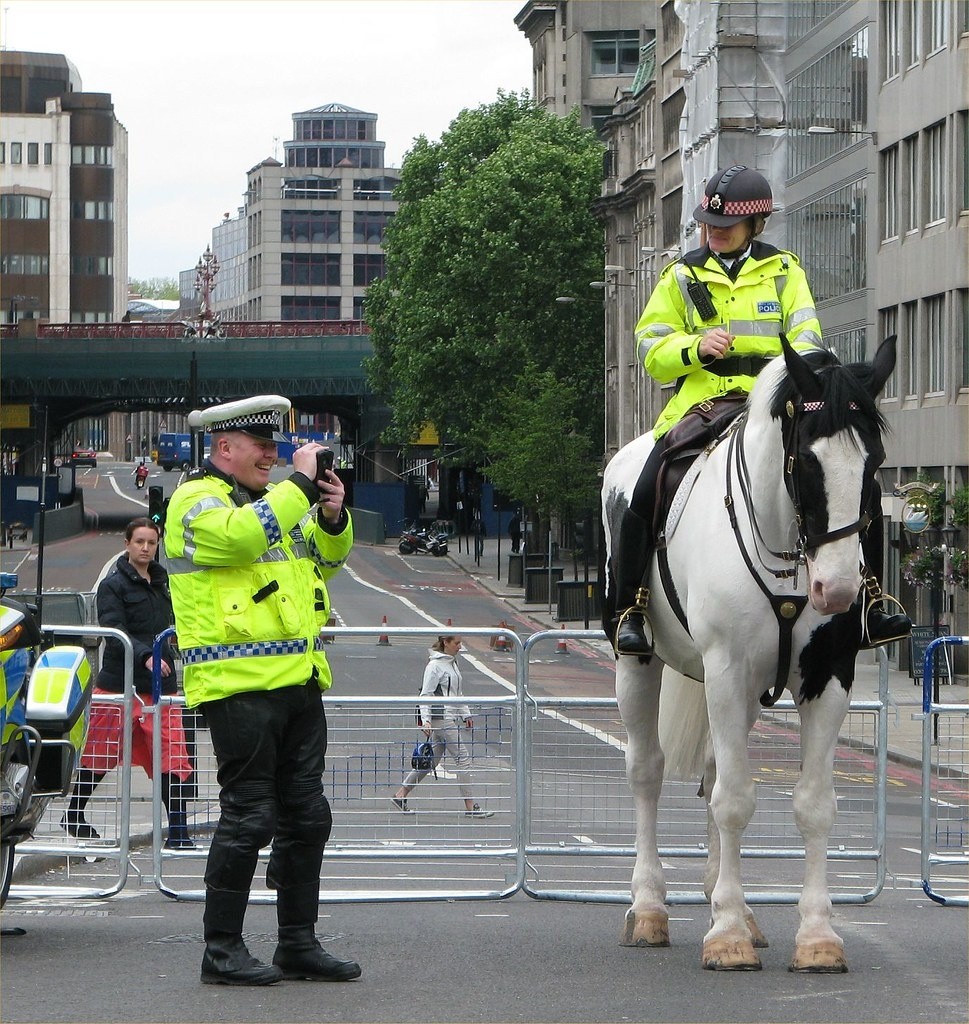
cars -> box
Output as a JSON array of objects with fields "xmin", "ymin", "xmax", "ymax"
[{"xmin": 73, "ymin": 450, "xmax": 97, "ymax": 467}]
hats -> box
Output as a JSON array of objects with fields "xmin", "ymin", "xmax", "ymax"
[{"xmin": 200, "ymin": 394, "xmax": 290, "ymax": 443}]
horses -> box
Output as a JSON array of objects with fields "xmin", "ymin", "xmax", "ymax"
[{"xmin": 601, "ymin": 332, "xmax": 898, "ymax": 974}]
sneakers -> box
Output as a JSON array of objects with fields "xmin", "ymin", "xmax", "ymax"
[
  {"xmin": 466, "ymin": 802, "xmax": 494, "ymax": 818},
  {"xmin": 392, "ymin": 795, "xmax": 415, "ymax": 815}
]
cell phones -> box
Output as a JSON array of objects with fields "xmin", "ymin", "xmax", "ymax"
[{"xmin": 315, "ymin": 449, "xmax": 334, "ymax": 488}]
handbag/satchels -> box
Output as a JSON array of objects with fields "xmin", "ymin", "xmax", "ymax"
[
  {"xmin": 414, "ymin": 680, "xmax": 445, "ymax": 719},
  {"xmin": 412, "ymin": 735, "xmax": 439, "ymax": 781}
]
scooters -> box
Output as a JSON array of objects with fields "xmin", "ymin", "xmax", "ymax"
[
  {"xmin": 135, "ymin": 466, "xmax": 148, "ymax": 489},
  {"xmin": 398, "ymin": 521, "xmax": 451, "ymax": 558}
]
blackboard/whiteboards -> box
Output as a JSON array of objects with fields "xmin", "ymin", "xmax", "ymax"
[{"xmin": 909, "ymin": 626, "xmax": 953, "ymax": 678}]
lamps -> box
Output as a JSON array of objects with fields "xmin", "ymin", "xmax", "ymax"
[
  {"xmin": 922, "ymin": 521, "xmax": 940, "ymax": 547},
  {"xmin": 590, "ymin": 281, "xmax": 637, "ymax": 291},
  {"xmin": 605, "ymin": 265, "xmax": 655, "ymax": 274},
  {"xmin": 903, "ymin": 527, "xmax": 920, "ymax": 547},
  {"xmin": 808, "ymin": 126, "xmax": 878, "ymax": 145},
  {"xmin": 641, "ymin": 246, "xmax": 682, "ymax": 254},
  {"xmin": 941, "ymin": 515, "xmax": 960, "ymax": 549},
  {"xmin": 555, "ymin": 297, "xmax": 608, "ymax": 308}
]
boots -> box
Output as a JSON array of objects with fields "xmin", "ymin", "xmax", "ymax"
[
  {"xmin": 272, "ymin": 879, "xmax": 361, "ymax": 982},
  {"xmin": 858, "ymin": 509, "xmax": 913, "ymax": 650},
  {"xmin": 610, "ymin": 508, "xmax": 650, "ymax": 653},
  {"xmin": 201, "ymin": 887, "xmax": 284, "ymax": 985}
]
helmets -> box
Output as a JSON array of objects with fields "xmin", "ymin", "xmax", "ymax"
[
  {"xmin": 693, "ymin": 165, "xmax": 773, "ymax": 227},
  {"xmin": 140, "ymin": 462, "xmax": 144, "ymax": 466}
]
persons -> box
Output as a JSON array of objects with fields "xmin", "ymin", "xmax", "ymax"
[
  {"xmin": 59, "ymin": 516, "xmax": 193, "ymax": 850},
  {"xmin": 163, "ymin": 394, "xmax": 362, "ymax": 985},
  {"xmin": 419, "ymin": 487, "xmax": 429, "ymax": 514},
  {"xmin": 613, "ymin": 163, "xmax": 912, "ymax": 652},
  {"xmin": 390, "ymin": 636, "xmax": 494, "ymax": 818},
  {"xmin": 122, "ymin": 311, "xmax": 131, "ymax": 327},
  {"xmin": 135, "ymin": 462, "xmax": 146, "ymax": 483},
  {"xmin": 469, "ymin": 519, "xmax": 487, "ymax": 556},
  {"xmin": 507, "ymin": 515, "xmax": 520, "ymax": 553}
]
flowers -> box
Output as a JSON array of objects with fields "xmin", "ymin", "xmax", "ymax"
[{"xmin": 898, "ymin": 544, "xmax": 969, "ymax": 591}]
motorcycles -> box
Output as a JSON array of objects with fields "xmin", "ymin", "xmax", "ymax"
[{"xmin": 1, "ymin": 443, "xmax": 97, "ymax": 913}]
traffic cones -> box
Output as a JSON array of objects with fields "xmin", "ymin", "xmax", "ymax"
[
  {"xmin": 493, "ymin": 622, "xmax": 510, "ymax": 652},
  {"xmin": 554, "ymin": 624, "xmax": 571, "ymax": 655},
  {"xmin": 446, "ymin": 619, "xmax": 451, "ymax": 627},
  {"xmin": 376, "ymin": 615, "xmax": 394, "ymax": 646}
]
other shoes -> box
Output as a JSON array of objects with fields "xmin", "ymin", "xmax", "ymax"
[
  {"xmin": 164, "ymin": 832, "xmax": 196, "ymax": 850},
  {"xmin": 59, "ymin": 809, "xmax": 99, "ymax": 839}
]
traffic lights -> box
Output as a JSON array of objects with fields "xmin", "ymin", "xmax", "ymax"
[
  {"xmin": 148, "ymin": 485, "xmax": 162, "ymax": 526},
  {"xmin": 575, "ymin": 520, "xmax": 592, "ymax": 549}
]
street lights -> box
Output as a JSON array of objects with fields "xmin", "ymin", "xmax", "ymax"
[
  {"xmin": 893, "ymin": 480, "xmax": 960, "ymax": 743},
  {"xmin": 194, "ymin": 244, "xmax": 220, "ymax": 321}
]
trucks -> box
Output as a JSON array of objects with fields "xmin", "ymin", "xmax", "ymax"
[{"xmin": 156, "ymin": 434, "xmax": 212, "ymax": 472}]
